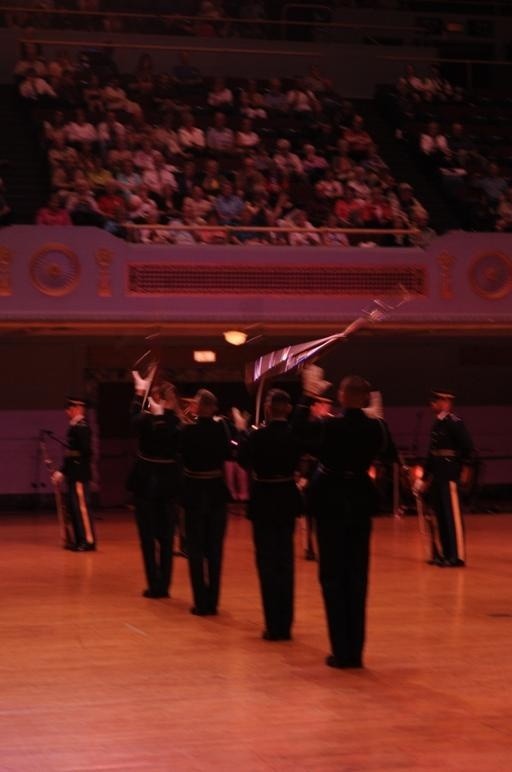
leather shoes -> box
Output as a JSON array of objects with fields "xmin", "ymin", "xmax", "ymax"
[
  {"xmin": 428, "ymin": 557, "xmax": 464, "ymax": 566},
  {"xmin": 65, "ymin": 544, "xmax": 94, "ymax": 551}
]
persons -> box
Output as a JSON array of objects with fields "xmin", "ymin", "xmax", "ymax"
[
  {"xmin": 41, "ymin": 393, "xmax": 98, "ymax": 551},
  {"xmin": 0, "ymin": 1, "xmax": 511, "ymax": 255},
  {"xmin": 143, "ymin": 388, "xmax": 241, "ymax": 616},
  {"xmin": 227, "ymin": 386, "xmax": 310, "ymax": 643},
  {"xmin": 126, "ymin": 368, "xmax": 185, "ymax": 600},
  {"xmin": 405, "ymin": 390, "xmax": 481, "ymax": 570},
  {"xmin": 290, "ymin": 363, "xmax": 399, "ymax": 669}
]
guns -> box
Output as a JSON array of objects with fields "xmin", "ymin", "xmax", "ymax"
[
  {"xmin": 40, "ymin": 441, "xmax": 74, "ymax": 550},
  {"xmin": 398, "ymin": 457, "xmax": 443, "ymax": 565}
]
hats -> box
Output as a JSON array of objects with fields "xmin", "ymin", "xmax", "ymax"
[
  {"xmin": 427, "ymin": 389, "xmax": 456, "ymax": 401},
  {"xmin": 65, "ymin": 400, "xmax": 85, "ymax": 407}
]
